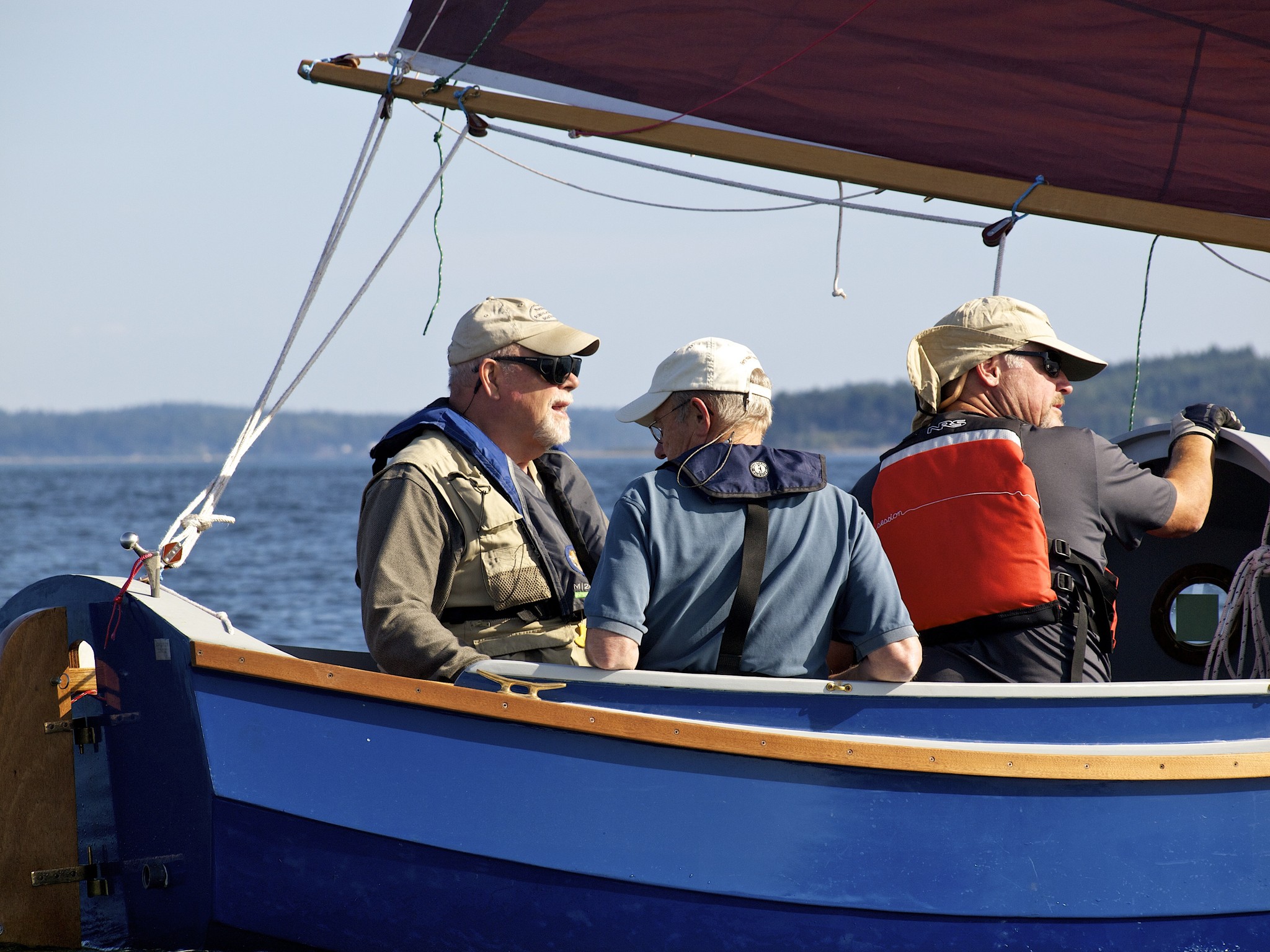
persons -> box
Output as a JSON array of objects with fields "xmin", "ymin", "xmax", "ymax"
[
  {"xmin": 583, "ymin": 338, "xmax": 922, "ymax": 682},
  {"xmin": 353, "ymin": 296, "xmax": 614, "ymax": 683},
  {"xmin": 847, "ymin": 296, "xmax": 1247, "ymax": 682}
]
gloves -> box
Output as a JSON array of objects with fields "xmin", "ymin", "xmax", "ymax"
[{"xmin": 1168, "ymin": 402, "xmax": 1245, "ymax": 459}]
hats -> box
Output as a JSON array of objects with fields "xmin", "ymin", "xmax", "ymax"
[
  {"xmin": 906, "ymin": 296, "xmax": 1109, "ymax": 433},
  {"xmin": 613, "ymin": 337, "xmax": 771, "ymax": 428},
  {"xmin": 447, "ymin": 295, "xmax": 600, "ymax": 367}
]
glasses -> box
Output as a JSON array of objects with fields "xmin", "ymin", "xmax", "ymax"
[
  {"xmin": 471, "ymin": 356, "xmax": 582, "ymax": 385},
  {"xmin": 1006, "ymin": 349, "xmax": 1059, "ymax": 377},
  {"xmin": 648, "ymin": 400, "xmax": 714, "ymax": 444}
]
